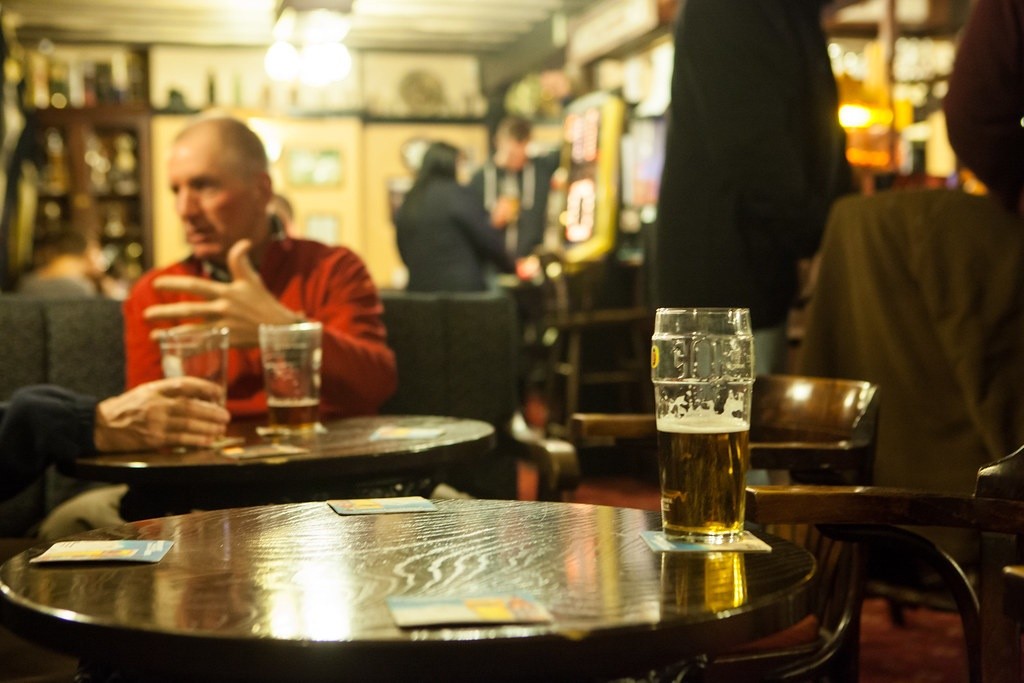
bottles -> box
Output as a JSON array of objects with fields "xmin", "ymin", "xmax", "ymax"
[{"xmin": 23, "ymin": 48, "xmax": 139, "ymax": 285}]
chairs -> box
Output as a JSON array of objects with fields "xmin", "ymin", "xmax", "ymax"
[{"xmin": 524, "ymin": 259, "xmax": 1024, "ymax": 683}]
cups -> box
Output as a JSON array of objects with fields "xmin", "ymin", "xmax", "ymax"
[
  {"xmin": 659, "ymin": 551, "xmax": 747, "ymax": 621},
  {"xmin": 258, "ymin": 322, "xmax": 322, "ymax": 445},
  {"xmin": 158, "ymin": 328, "xmax": 230, "ymax": 444},
  {"xmin": 650, "ymin": 307, "xmax": 756, "ymax": 546}
]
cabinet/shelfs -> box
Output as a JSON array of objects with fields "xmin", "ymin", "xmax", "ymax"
[{"xmin": 22, "ymin": 102, "xmax": 156, "ymax": 303}]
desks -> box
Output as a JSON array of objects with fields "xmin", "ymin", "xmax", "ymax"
[
  {"xmin": 0, "ymin": 497, "xmax": 818, "ymax": 683},
  {"xmin": 71, "ymin": 415, "xmax": 496, "ymax": 521}
]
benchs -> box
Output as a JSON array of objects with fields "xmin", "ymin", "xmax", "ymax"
[{"xmin": 0, "ymin": 290, "xmax": 518, "ymax": 541}]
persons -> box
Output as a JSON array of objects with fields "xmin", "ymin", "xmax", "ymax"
[
  {"xmin": 397, "ymin": 143, "xmax": 530, "ymax": 293},
  {"xmin": 0, "ymin": 377, "xmax": 230, "ymax": 498},
  {"xmin": 467, "ymin": 120, "xmax": 550, "ymax": 272},
  {"xmin": 0, "ymin": 227, "xmax": 102, "ymax": 297},
  {"xmin": 122, "ymin": 117, "xmax": 395, "ymax": 518}
]
